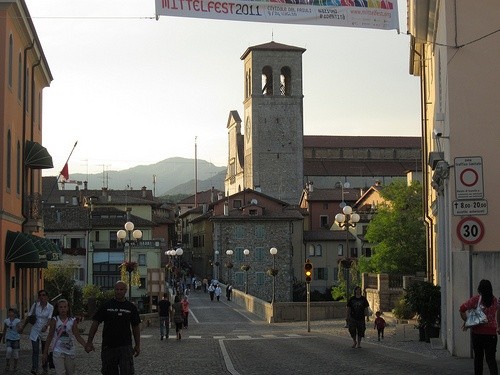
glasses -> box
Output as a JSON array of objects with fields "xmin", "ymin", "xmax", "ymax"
[{"xmin": 10, "ymin": 323, "xmax": 12, "ymax": 329}]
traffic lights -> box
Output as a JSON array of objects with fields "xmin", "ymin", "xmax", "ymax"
[{"xmin": 305, "ymin": 262, "xmax": 313, "ymax": 283}]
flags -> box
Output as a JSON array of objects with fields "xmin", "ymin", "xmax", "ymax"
[{"xmin": 61, "ymin": 162, "xmax": 69, "ymax": 180}]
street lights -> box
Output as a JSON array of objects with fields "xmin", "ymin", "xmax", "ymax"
[
  {"xmin": 215, "ymin": 250, "xmax": 220, "ymax": 280},
  {"xmin": 226, "ymin": 250, "xmax": 233, "ymax": 284},
  {"xmin": 243, "ymin": 248, "xmax": 250, "ymax": 293},
  {"xmin": 165, "ymin": 248, "xmax": 183, "ymax": 293},
  {"xmin": 270, "ymin": 247, "xmax": 277, "ymax": 303},
  {"xmin": 335, "ymin": 205, "xmax": 360, "ymax": 328},
  {"xmin": 116, "ymin": 221, "xmax": 143, "ymax": 302}
]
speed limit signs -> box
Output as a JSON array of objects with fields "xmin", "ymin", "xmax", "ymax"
[{"xmin": 457, "ymin": 216, "xmax": 486, "ymax": 244}]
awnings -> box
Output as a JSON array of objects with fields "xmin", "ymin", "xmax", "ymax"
[
  {"xmin": 25, "ymin": 142, "xmax": 53, "ymax": 169},
  {"xmin": 5, "ymin": 230, "xmax": 63, "ymax": 268}
]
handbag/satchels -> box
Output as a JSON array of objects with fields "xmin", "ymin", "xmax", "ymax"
[
  {"xmin": 28, "ymin": 302, "xmax": 37, "ymax": 324},
  {"xmin": 464, "ymin": 295, "xmax": 487, "ymax": 327},
  {"xmin": 48, "ymin": 352, "xmax": 55, "ymax": 369}
]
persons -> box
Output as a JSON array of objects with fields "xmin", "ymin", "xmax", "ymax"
[
  {"xmin": 458, "ymin": 278, "xmax": 500, "ymax": 375},
  {"xmin": 225, "ymin": 283, "xmax": 232, "ymax": 301},
  {"xmin": 374, "ymin": 311, "xmax": 386, "ymax": 341},
  {"xmin": 177, "ymin": 275, "xmax": 221, "ymax": 303},
  {"xmin": 172, "ymin": 295, "xmax": 185, "ymax": 340},
  {"xmin": 346, "ymin": 286, "xmax": 370, "ymax": 348},
  {"xmin": 157, "ymin": 293, "xmax": 172, "ymax": 340},
  {"xmin": 3, "ymin": 290, "xmax": 87, "ymax": 375},
  {"xmin": 182, "ymin": 297, "xmax": 189, "ymax": 329},
  {"xmin": 84, "ymin": 280, "xmax": 141, "ymax": 375}
]
